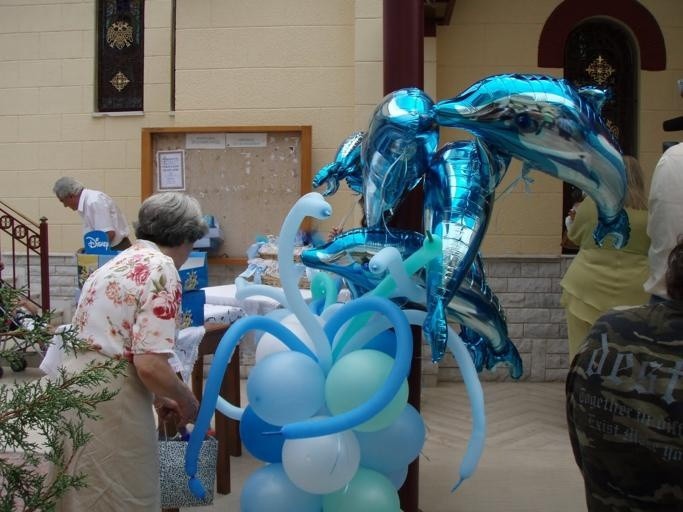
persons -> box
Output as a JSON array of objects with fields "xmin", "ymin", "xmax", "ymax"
[
  {"xmin": 5, "ymin": 297, "xmax": 56, "ymax": 353},
  {"xmin": 565, "ymin": 231, "xmax": 683, "ymax": 512},
  {"xmin": 560, "ymin": 155, "xmax": 650, "ymax": 364},
  {"xmin": 60, "ymin": 192, "xmax": 209, "ymax": 512},
  {"xmin": 53, "ymin": 177, "xmax": 131, "ymax": 249},
  {"xmin": 642, "ymin": 142, "xmax": 683, "ymax": 303}
]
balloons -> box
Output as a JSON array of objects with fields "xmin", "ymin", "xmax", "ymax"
[
  {"xmin": 432, "ymin": 74, "xmax": 631, "ymax": 250},
  {"xmin": 186, "ymin": 88, "xmax": 524, "ymax": 511}
]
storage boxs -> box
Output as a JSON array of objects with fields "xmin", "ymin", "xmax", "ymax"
[{"xmin": 76, "ymin": 215, "xmax": 223, "ymax": 330}]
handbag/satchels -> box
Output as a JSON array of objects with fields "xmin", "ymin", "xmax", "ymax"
[{"xmin": 157, "ymin": 434, "xmax": 220, "ymax": 509}]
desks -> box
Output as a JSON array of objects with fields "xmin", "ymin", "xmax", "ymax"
[{"xmin": 159, "ymin": 328, "xmax": 240, "ymax": 494}]
